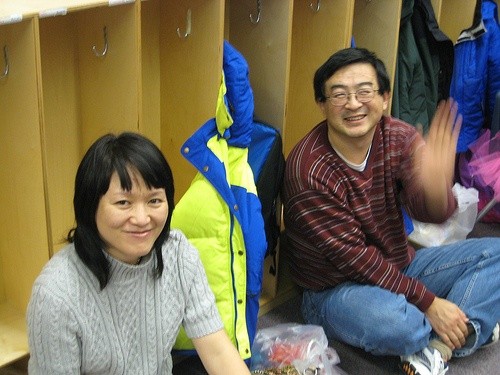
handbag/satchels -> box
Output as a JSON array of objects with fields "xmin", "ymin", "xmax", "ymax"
[{"xmin": 249, "ymin": 322, "xmax": 346, "ymax": 375}]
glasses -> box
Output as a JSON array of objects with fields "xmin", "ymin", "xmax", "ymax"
[{"xmin": 325, "ymin": 88, "xmax": 380, "ymax": 105}]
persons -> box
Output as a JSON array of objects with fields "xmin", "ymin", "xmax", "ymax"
[
  {"xmin": 281, "ymin": 43, "xmax": 500, "ymax": 375},
  {"xmin": 26, "ymin": 129, "xmax": 255, "ymax": 375}
]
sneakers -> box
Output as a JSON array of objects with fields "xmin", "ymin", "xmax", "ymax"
[{"xmin": 401, "ymin": 343, "xmax": 448, "ymax": 375}]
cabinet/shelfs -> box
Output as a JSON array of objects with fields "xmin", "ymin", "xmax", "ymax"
[{"xmin": 0, "ymin": 0, "xmax": 402, "ymax": 366}]
